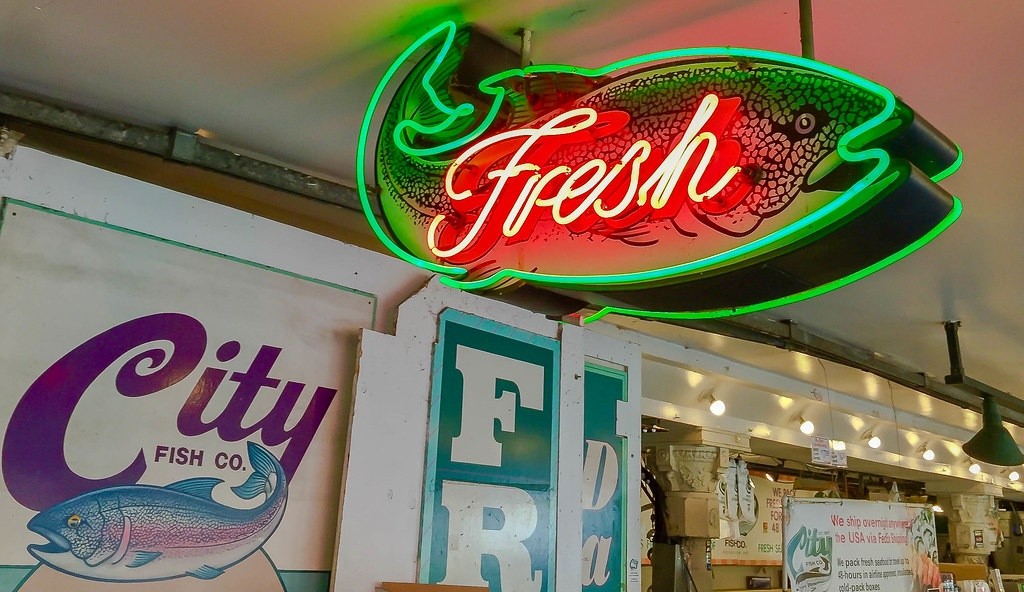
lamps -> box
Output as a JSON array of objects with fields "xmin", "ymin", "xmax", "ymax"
[
  {"xmin": 765, "ymin": 461, "xmax": 784, "ymax": 482},
  {"xmin": 961, "ymin": 396, "xmax": 1024, "ymax": 467}
]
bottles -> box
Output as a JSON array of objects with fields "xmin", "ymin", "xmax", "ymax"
[{"xmin": 888, "ymin": 480, "xmax": 901, "ymax": 503}]
